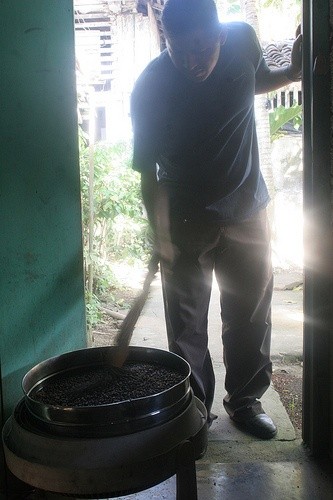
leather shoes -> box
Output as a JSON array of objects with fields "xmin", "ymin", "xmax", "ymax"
[{"xmin": 239, "ymin": 412, "xmax": 277, "ymax": 438}]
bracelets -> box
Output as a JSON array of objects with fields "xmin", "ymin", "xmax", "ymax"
[{"xmin": 285, "ymin": 65, "xmax": 302, "ymax": 82}]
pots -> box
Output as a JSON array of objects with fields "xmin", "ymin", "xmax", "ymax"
[{"xmin": 20, "ymin": 346, "xmax": 192, "ymax": 438}]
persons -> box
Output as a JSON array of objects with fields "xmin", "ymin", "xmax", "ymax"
[{"xmin": 130, "ymin": 0, "xmax": 304, "ymax": 462}]
